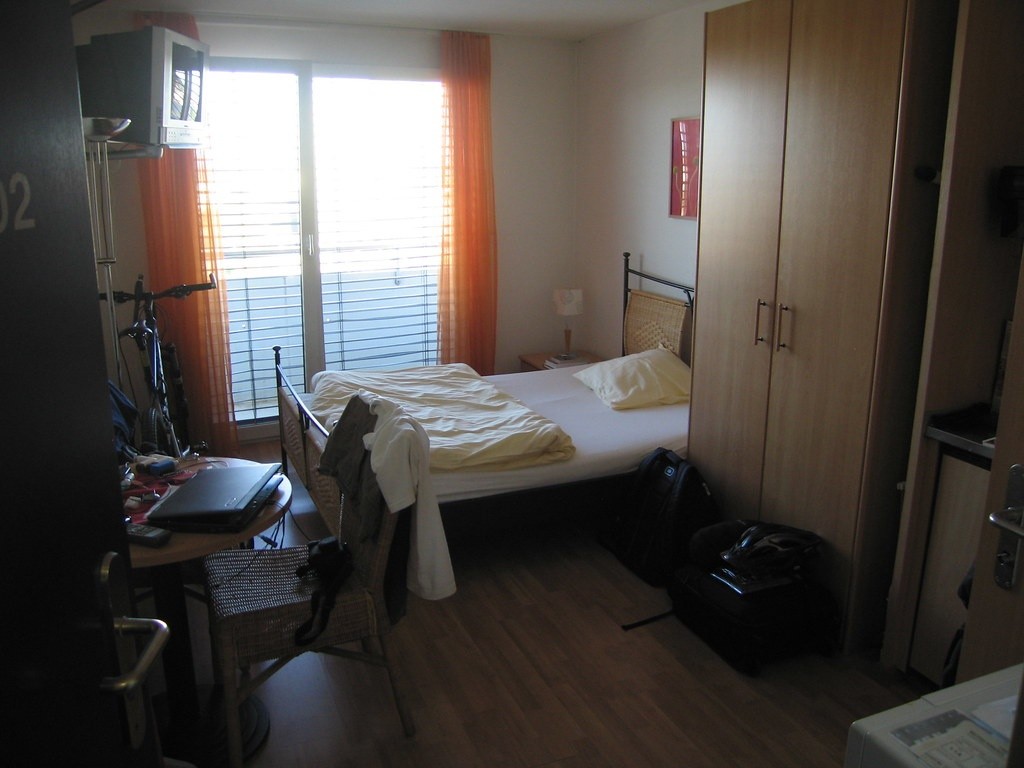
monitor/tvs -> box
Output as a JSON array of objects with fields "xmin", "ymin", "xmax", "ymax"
[{"xmin": 74, "ymin": 25, "xmax": 209, "ymax": 150}]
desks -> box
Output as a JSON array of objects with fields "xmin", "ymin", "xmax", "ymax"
[{"xmin": 121, "ymin": 456, "xmax": 293, "ymax": 767}]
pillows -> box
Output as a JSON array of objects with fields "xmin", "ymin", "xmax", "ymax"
[{"xmin": 573, "ymin": 347, "xmax": 692, "ymax": 410}]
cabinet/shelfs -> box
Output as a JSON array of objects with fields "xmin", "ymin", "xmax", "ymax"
[
  {"xmin": 881, "ymin": 1, "xmax": 1023, "ymax": 687},
  {"xmin": 690, "ymin": 0, "xmax": 956, "ymax": 658}
]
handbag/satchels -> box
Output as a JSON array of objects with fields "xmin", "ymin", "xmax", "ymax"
[{"xmin": 295, "ymin": 537, "xmax": 357, "ymax": 649}]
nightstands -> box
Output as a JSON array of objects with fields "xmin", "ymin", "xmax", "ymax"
[{"xmin": 517, "ymin": 349, "xmax": 609, "ymax": 372}]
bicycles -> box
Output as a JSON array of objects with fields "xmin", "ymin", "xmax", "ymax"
[{"xmin": 98, "ymin": 271, "xmax": 218, "ymax": 458}]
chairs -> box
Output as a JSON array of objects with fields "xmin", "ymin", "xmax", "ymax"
[{"xmin": 200, "ymin": 387, "xmax": 423, "ymax": 768}]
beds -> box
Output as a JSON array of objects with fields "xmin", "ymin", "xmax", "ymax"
[{"xmin": 274, "ymin": 252, "xmax": 693, "ymax": 623}]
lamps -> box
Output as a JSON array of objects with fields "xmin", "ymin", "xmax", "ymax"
[
  {"xmin": 553, "ymin": 285, "xmax": 584, "ymax": 361},
  {"xmin": 83, "ymin": 114, "xmax": 149, "ymax": 454}
]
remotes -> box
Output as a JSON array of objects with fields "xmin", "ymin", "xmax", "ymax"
[{"xmin": 125, "ymin": 523, "xmax": 172, "ymax": 547}]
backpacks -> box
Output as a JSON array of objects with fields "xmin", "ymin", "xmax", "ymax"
[{"xmin": 597, "ymin": 447, "xmax": 720, "ymax": 589}]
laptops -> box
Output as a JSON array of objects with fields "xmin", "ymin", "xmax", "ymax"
[{"xmin": 146, "ymin": 463, "xmax": 283, "ymax": 533}]
